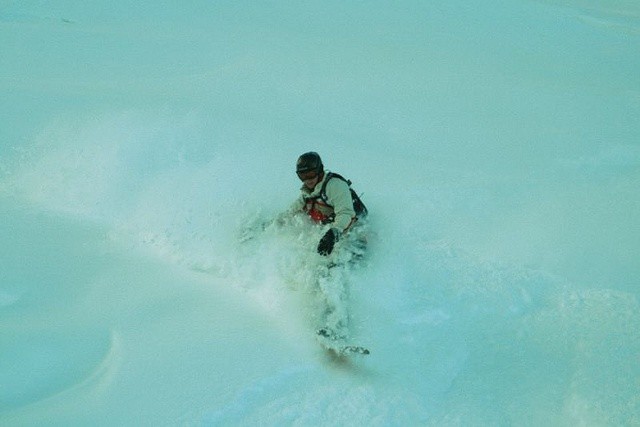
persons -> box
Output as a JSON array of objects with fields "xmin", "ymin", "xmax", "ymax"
[{"xmin": 254, "ymin": 151, "xmax": 370, "ymax": 356}]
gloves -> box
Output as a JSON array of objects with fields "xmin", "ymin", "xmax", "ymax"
[{"xmin": 317, "ymin": 229, "xmax": 336, "ymax": 257}]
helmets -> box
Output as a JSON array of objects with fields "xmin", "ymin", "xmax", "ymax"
[{"xmin": 296, "ymin": 151, "xmax": 324, "ymax": 191}]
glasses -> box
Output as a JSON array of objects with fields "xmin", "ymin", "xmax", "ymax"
[{"xmin": 297, "ymin": 161, "xmax": 320, "ymax": 181}]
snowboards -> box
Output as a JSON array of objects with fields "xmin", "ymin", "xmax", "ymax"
[{"xmin": 316, "ymin": 331, "xmax": 371, "ymax": 357}]
